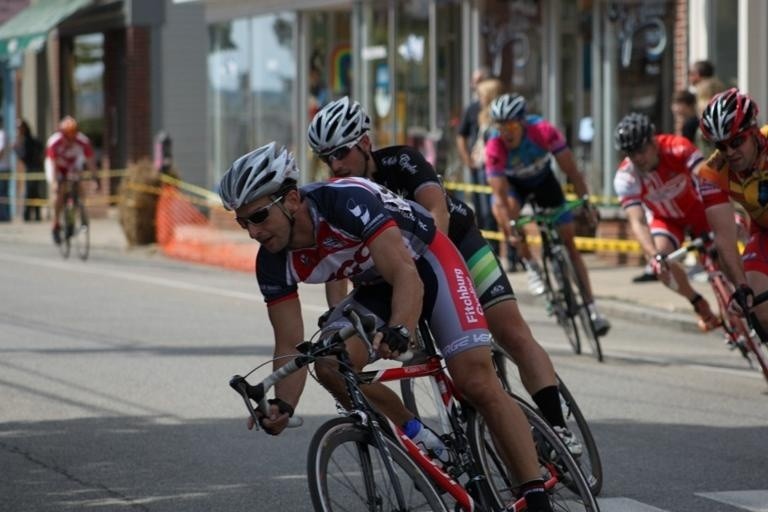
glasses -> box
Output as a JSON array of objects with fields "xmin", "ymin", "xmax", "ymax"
[
  {"xmin": 318, "ymin": 130, "xmax": 368, "ymax": 163},
  {"xmin": 237, "ymin": 197, "xmax": 285, "ymax": 229},
  {"xmin": 715, "ymin": 128, "xmax": 753, "ymax": 152}
]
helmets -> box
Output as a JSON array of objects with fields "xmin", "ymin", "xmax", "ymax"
[
  {"xmin": 700, "ymin": 89, "xmax": 758, "ymax": 142},
  {"xmin": 614, "ymin": 113, "xmax": 654, "ymax": 152},
  {"xmin": 60, "ymin": 116, "xmax": 78, "ymax": 138},
  {"xmin": 488, "ymin": 93, "xmax": 526, "ymax": 121},
  {"xmin": 307, "ymin": 96, "xmax": 370, "ymax": 154},
  {"xmin": 219, "ymin": 141, "xmax": 299, "ymax": 212}
]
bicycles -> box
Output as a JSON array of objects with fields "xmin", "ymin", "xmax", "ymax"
[
  {"xmin": 229, "ymin": 307, "xmax": 598, "ymax": 511},
  {"xmin": 49, "ymin": 174, "xmax": 104, "ymax": 263},
  {"xmin": 729, "ymin": 281, "xmax": 767, "ymax": 350},
  {"xmin": 374, "ymin": 313, "xmax": 606, "ymax": 495},
  {"xmin": 506, "ymin": 201, "xmax": 611, "ymax": 362},
  {"xmin": 649, "ymin": 223, "xmax": 767, "ymax": 385}
]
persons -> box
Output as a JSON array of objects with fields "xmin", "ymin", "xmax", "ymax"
[
  {"xmin": 305, "ymin": 95, "xmax": 585, "ymax": 464},
  {"xmin": 9, "ymin": 118, "xmax": 42, "ymax": 221},
  {"xmin": 218, "ymin": 138, "xmax": 550, "ymax": 511},
  {"xmin": 0, "ymin": 113, "xmax": 7, "ymax": 221},
  {"xmin": 43, "ymin": 112, "xmax": 103, "ymax": 244}
]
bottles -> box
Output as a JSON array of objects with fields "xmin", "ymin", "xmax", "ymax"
[
  {"xmin": 455, "ymin": 430, "xmax": 482, "ymax": 484},
  {"xmin": 401, "ymin": 418, "xmax": 455, "ymax": 470}
]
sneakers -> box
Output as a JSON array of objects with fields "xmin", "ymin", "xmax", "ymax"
[
  {"xmin": 691, "ymin": 297, "xmax": 720, "ymax": 331},
  {"xmin": 590, "ymin": 312, "xmax": 610, "ymax": 334},
  {"xmin": 414, "ymin": 444, "xmax": 458, "ymax": 492},
  {"xmin": 552, "ymin": 427, "xmax": 583, "ymax": 461},
  {"xmin": 525, "ymin": 262, "xmax": 548, "ymax": 294}
]
